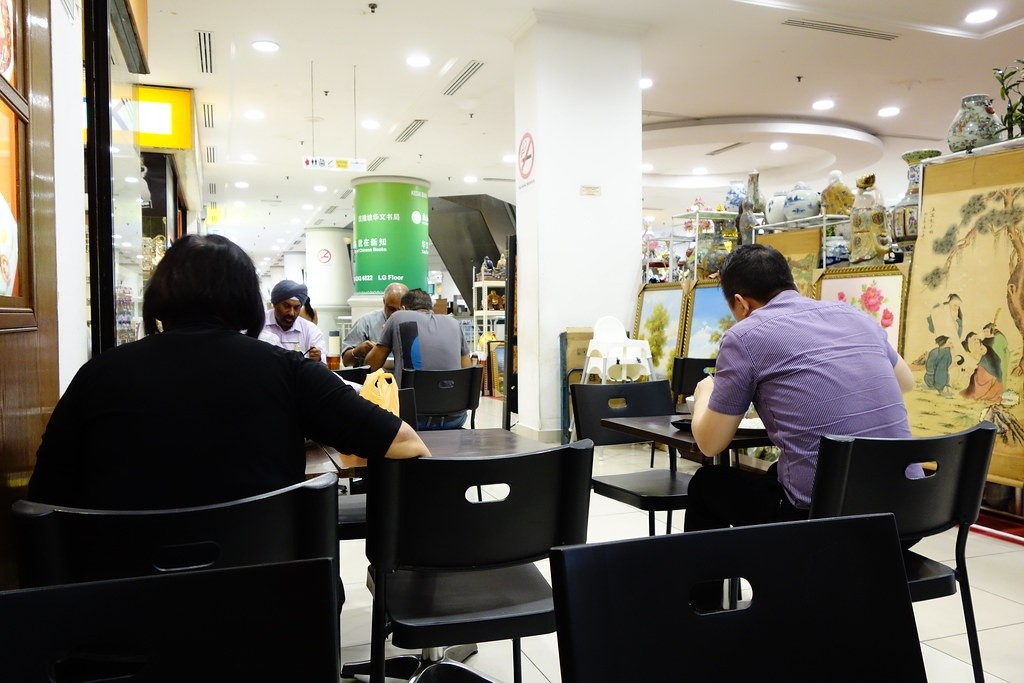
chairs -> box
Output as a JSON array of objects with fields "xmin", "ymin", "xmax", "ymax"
[{"xmin": 0, "ymin": 356, "xmax": 1000, "ymax": 683}]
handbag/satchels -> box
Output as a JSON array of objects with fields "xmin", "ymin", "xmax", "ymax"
[{"xmin": 359, "ymin": 368, "xmax": 400, "ymax": 416}]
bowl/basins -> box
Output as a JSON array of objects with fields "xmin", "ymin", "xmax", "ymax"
[{"xmin": 383, "ymin": 357, "xmax": 395, "ymax": 371}]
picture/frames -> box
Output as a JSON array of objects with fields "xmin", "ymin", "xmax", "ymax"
[
  {"xmin": 633, "ymin": 281, "xmax": 689, "ymax": 384},
  {"xmin": 489, "ymin": 342, "xmax": 504, "ymax": 398},
  {"xmin": 811, "ymin": 263, "xmax": 910, "ymax": 358},
  {"xmin": 683, "ymin": 279, "xmax": 737, "ymax": 360}
]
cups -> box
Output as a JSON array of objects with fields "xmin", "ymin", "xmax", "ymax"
[
  {"xmin": 685, "ymin": 396, "xmax": 694, "ymax": 416},
  {"xmin": 326, "ymin": 354, "xmax": 340, "ymax": 370}
]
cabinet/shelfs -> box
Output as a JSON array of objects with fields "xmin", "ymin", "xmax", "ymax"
[
  {"xmin": 646, "ymin": 237, "xmax": 694, "ymax": 281},
  {"xmin": 471, "ymin": 266, "xmax": 506, "ymax": 396},
  {"xmin": 668, "ymin": 213, "xmax": 739, "ymax": 280}
]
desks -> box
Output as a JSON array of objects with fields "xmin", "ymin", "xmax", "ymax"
[
  {"xmin": 601, "ymin": 413, "xmax": 774, "ymax": 467},
  {"xmin": 306, "ymin": 429, "xmax": 552, "ymax": 683}
]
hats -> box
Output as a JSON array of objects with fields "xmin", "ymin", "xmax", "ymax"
[{"xmin": 271, "ymin": 280, "xmax": 308, "ymax": 306}]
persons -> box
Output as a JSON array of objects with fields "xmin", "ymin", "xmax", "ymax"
[
  {"xmin": 341, "ymin": 283, "xmax": 409, "ymax": 369},
  {"xmin": 483, "ymin": 254, "xmax": 507, "ymax": 272},
  {"xmin": 488, "ymin": 289, "xmax": 503, "ymax": 310},
  {"xmin": 683, "ymin": 244, "xmax": 925, "ymax": 614},
  {"xmin": 366, "ymin": 288, "xmax": 472, "ymax": 430},
  {"xmin": 241, "ymin": 280, "xmax": 328, "ymax": 365},
  {"xmin": 26, "ymin": 232, "xmax": 432, "ymax": 614}
]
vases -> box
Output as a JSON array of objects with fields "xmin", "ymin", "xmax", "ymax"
[
  {"xmin": 702, "ymin": 218, "xmax": 730, "ymax": 279},
  {"xmin": 890, "ymin": 149, "xmax": 943, "ymax": 263},
  {"xmin": 946, "ymin": 94, "xmax": 1003, "ymax": 153}
]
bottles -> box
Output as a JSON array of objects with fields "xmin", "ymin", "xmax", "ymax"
[
  {"xmin": 724, "ymin": 180, "xmax": 746, "ymax": 212},
  {"xmin": 891, "ymin": 149, "xmax": 941, "ymax": 263},
  {"xmin": 821, "ymin": 236, "xmax": 850, "ymax": 268},
  {"xmin": 118, "ymin": 320, "xmax": 130, "ymax": 329},
  {"xmin": 329, "ymin": 331, "xmax": 340, "ymax": 357},
  {"xmin": 687, "ymin": 219, "xmax": 730, "ymax": 278},
  {"xmin": 765, "ymin": 181, "xmax": 821, "ymax": 233},
  {"xmin": 947, "ymin": 94, "xmax": 1004, "ymax": 154}
]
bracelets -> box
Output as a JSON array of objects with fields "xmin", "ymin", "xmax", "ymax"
[{"xmin": 352, "ymin": 348, "xmax": 359, "ymax": 359}]
